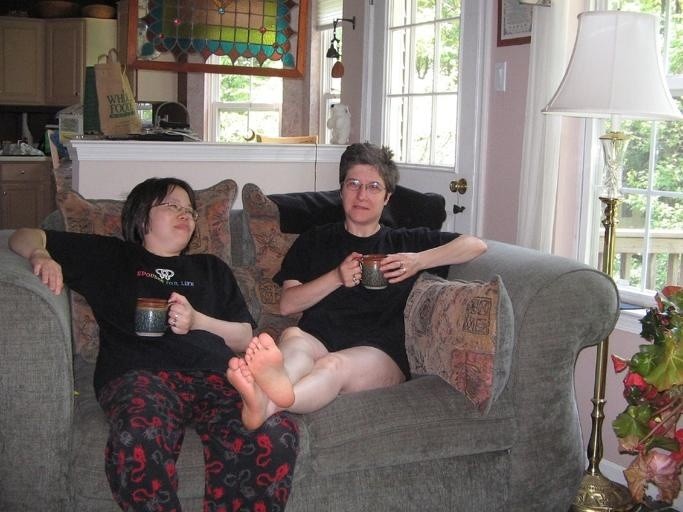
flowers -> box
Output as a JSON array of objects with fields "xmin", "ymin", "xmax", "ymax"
[{"xmin": 612, "ymin": 287, "xmax": 683, "ymax": 512}]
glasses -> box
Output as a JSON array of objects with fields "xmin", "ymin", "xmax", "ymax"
[
  {"xmin": 151, "ymin": 202, "xmax": 199, "ymax": 221},
  {"xmin": 339, "ymin": 178, "xmax": 387, "ymax": 194}
]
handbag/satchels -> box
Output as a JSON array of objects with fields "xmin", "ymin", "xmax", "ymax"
[{"xmin": 83, "ymin": 48, "xmax": 142, "ymax": 138}]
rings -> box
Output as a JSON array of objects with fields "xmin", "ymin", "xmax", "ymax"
[
  {"xmin": 355, "ymin": 280, "xmax": 360, "ymax": 286},
  {"xmin": 174, "ymin": 314, "xmax": 178, "ymax": 321},
  {"xmin": 173, "ymin": 321, "xmax": 177, "ymax": 327},
  {"xmin": 400, "ymin": 261, "xmax": 404, "ymax": 269},
  {"xmin": 402, "ymin": 268, "xmax": 407, "ymax": 275},
  {"xmin": 352, "ymin": 274, "xmax": 357, "ymax": 282}
]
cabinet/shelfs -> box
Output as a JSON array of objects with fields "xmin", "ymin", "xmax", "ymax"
[
  {"xmin": 1, "ymin": 16, "xmax": 47, "ymax": 107},
  {"xmin": 46, "ymin": 18, "xmax": 118, "ymax": 106},
  {"xmin": 1, "ymin": 157, "xmax": 52, "ymax": 229}
]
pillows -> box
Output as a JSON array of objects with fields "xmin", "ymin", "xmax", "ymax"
[
  {"xmin": 242, "ymin": 183, "xmax": 298, "ymax": 281},
  {"xmin": 402, "ymin": 271, "xmax": 513, "ymax": 417},
  {"xmin": 231, "ymin": 265, "xmax": 303, "ymax": 358},
  {"xmin": 56, "ymin": 179, "xmax": 238, "ymax": 362}
]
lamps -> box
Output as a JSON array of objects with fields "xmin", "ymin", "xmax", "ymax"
[
  {"xmin": 541, "ymin": 11, "xmax": 683, "ymax": 512},
  {"xmin": 326, "ymin": 16, "xmax": 356, "ymax": 79}
]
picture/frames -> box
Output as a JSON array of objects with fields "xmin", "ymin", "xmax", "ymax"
[
  {"xmin": 496, "ymin": 0, "xmax": 532, "ymax": 48},
  {"xmin": 127, "ymin": 0, "xmax": 309, "ymax": 78}
]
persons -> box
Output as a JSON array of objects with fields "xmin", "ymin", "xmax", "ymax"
[
  {"xmin": 226, "ymin": 143, "xmax": 487, "ymax": 431},
  {"xmin": 8, "ymin": 179, "xmax": 300, "ymax": 512}
]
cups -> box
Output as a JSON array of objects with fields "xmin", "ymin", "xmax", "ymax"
[
  {"xmin": 134, "ymin": 298, "xmax": 175, "ymax": 337},
  {"xmin": 353, "ymin": 253, "xmax": 389, "ymax": 291}
]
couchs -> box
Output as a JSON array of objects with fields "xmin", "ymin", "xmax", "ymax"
[{"xmin": 0, "ymin": 209, "xmax": 620, "ymax": 511}]
